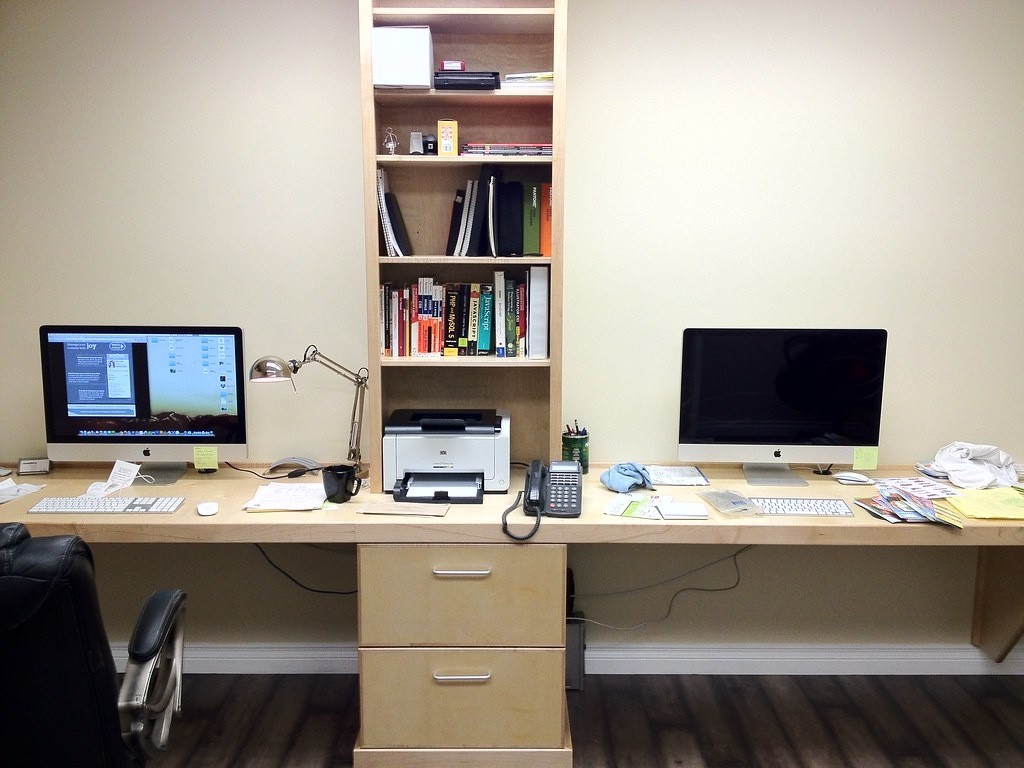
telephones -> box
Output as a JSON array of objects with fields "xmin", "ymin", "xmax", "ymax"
[{"xmin": 524, "ymin": 459, "xmax": 582, "ymax": 518}]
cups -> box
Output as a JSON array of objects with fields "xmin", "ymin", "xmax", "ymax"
[
  {"xmin": 322, "ymin": 465, "xmax": 361, "ymax": 504},
  {"xmin": 563, "ymin": 432, "xmax": 590, "ymax": 475}
]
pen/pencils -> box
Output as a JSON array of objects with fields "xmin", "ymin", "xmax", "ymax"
[{"xmin": 565, "ymin": 420, "xmax": 588, "ymax": 436}]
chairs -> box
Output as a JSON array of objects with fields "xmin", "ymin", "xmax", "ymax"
[{"xmin": 0, "ymin": 523, "xmax": 188, "ymax": 767}]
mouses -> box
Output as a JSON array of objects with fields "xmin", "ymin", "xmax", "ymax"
[
  {"xmin": 831, "ymin": 471, "xmax": 868, "ymax": 482},
  {"xmin": 197, "ymin": 502, "xmax": 219, "ymax": 516}
]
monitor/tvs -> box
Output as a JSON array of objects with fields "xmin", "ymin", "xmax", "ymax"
[
  {"xmin": 38, "ymin": 324, "xmax": 248, "ymax": 487},
  {"xmin": 677, "ymin": 327, "xmax": 887, "ymax": 487}
]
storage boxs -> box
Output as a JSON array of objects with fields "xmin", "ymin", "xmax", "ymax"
[{"xmin": 372, "ymin": 25, "xmax": 434, "ymax": 90}]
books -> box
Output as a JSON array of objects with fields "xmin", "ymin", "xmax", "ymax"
[
  {"xmin": 500, "ymin": 71, "xmax": 554, "ymax": 89},
  {"xmin": 380, "ymin": 267, "xmax": 548, "ymax": 360},
  {"xmin": 377, "ymin": 167, "xmax": 413, "ymax": 257},
  {"xmin": 459, "ymin": 142, "xmax": 553, "ymax": 156},
  {"xmin": 912, "ymin": 462, "xmax": 950, "ymax": 483},
  {"xmin": 446, "ymin": 164, "xmax": 552, "ymax": 258}
]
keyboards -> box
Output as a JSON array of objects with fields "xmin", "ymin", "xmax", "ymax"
[
  {"xmin": 26, "ymin": 497, "xmax": 185, "ymax": 514},
  {"xmin": 749, "ymin": 497, "xmax": 855, "ymax": 516}
]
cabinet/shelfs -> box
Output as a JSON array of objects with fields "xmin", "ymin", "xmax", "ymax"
[
  {"xmin": 358, "ymin": 0, "xmax": 567, "ymax": 469},
  {"xmin": 0, "ymin": 460, "xmax": 1024, "ymax": 768}
]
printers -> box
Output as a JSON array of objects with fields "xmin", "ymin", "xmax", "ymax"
[{"xmin": 383, "ymin": 408, "xmax": 509, "ymax": 505}]
